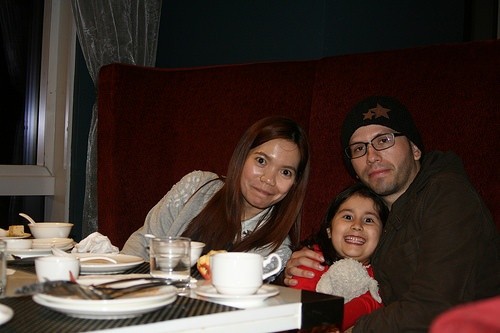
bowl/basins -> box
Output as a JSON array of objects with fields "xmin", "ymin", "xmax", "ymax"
[
  {"xmin": 160, "ymin": 241, "xmax": 205, "ymax": 268},
  {"xmin": 28, "ymin": 223, "xmax": 74, "ymax": 238},
  {"xmin": 157, "ymin": 254, "xmax": 180, "ymax": 272}
]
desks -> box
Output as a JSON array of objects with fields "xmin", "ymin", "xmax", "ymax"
[{"xmin": 0, "ymin": 248, "xmax": 344, "ymax": 333}]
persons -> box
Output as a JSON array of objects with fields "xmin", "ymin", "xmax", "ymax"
[
  {"xmin": 289, "ymin": 182, "xmax": 386, "ymax": 330},
  {"xmin": 274, "ymin": 95, "xmax": 500, "ymax": 333},
  {"xmin": 119, "ymin": 115, "xmax": 311, "ymax": 283}
]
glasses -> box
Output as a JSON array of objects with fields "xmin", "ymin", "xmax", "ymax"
[{"xmin": 344, "ymin": 132, "xmax": 406, "ymax": 159}]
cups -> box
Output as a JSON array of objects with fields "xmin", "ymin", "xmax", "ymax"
[
  {"xmin": 35, "ymin": 256, "xmax": 79, "ymax": 283},
  {"xmin": 210, "ymin": 253, "xmax": 282, "ymax": 294},
  {"xmin": 0, "ymin": 239, "xmax": 7, "ymax": 293},
  {"xmin": 149, "ymin": 237, "xmax": 191, "ymax": 280},
  {"xmin": 8, "ymin": 239, "xmax": 33, "ymax": 249}
]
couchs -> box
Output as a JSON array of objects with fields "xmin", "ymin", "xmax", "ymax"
[{"xmin": 98, "ymin": 39, "xmax": 500, "ymax": 333}]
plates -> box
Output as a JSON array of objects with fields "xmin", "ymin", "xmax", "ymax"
[
  {"xmin": 0, "ymin": 304, "xmax": 13, "ymax": 325},
  {"xmin": 6, "ymin": 238, "xmax": 74, "ymax": 264},
  {"xmin": 195, "ymin": 284, "xmax": 281, "ymax": 302},
  {"xmin": 33, "ymin": 279, "xmax": 178, "ymax": 321},
  {"xmin": 0, "ymin": 231, "xmax": 31, "ymax": 239},
  {"xmin": 72, "ymin": 254, "xmax": 144, "ymax": 274}
]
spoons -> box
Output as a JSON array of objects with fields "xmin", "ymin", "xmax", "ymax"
[{"xmin": 52, "ymin": 247, "xmax": 116, "ymax": 264}]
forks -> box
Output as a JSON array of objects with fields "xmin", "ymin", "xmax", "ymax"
[{"xmin": 76, "ymin": 278, "xmax": 190, "ymax": 300}]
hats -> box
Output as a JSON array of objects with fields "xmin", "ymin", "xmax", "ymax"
[{"xmin": 340, "ymin": 95, "xmax": 422, "ymax": 180}]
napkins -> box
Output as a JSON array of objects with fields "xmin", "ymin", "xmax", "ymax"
[{"xmin": 71, "ymin": 231, "xmax": 121, "ymax": 254}]
